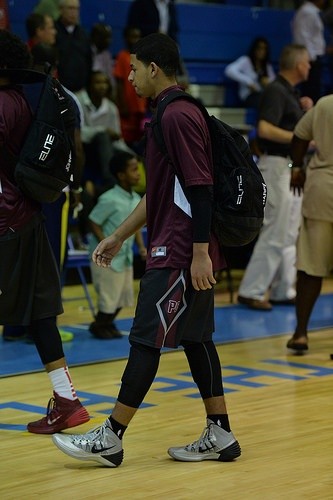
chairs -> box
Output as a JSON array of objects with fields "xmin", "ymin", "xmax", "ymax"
[{"xmin": 61, "ymin": 232, "xmax": 96, "ymax": 318}]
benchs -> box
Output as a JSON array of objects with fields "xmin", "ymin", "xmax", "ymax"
[{"xmin": 8, "ymin": 0, "xmax": 333, "ymax": 147}]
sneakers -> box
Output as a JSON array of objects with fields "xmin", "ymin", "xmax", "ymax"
[
  {"xmin": 52, "ymin": 425, "xmax": 123, "ymax": 467},
  {"xmin": 168, "ymin": 422, "xmax": 241, "ymax": 462},
  {"xmin": 27, "ymin": 391, "xmax": 90, "ymax": 434}
]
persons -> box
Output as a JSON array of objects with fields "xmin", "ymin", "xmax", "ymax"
[
  {"xmin": 226, "ymin": 37, "xmax": 277, "ymax": 104},
  {"xmin": 84, "ymin": 152, "xmax": 149, "ymax": 339},
  {"xmin": 0, "ymin": 19, "xmax": 90, "ymax": 434},
  {"xmin": 287, "ymin": 93, "xmax": 333, "ymax": 361},
  {"xmin": 0, "ymin": 0, "xmax": 180, "ymax": 250},
  {"xmin": 51, "ymin": 30, "xmax": 241, "ymax": 468},
  {"xmin": 288, "ymin": 0, "xmax": 326, "ymax": 107},
  {"xmin": 232, "ymin": 44, "xmax": 314, "ymax": 312}
]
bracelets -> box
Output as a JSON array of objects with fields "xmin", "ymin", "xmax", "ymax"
[{"xmin": 287, "ymin": 162, "xmax": 304, "ymax": 168}]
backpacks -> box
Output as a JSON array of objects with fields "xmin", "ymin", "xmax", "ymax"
[
  {"xmin": 150, "ymin": 90, "xmax": 267, "ymax": 247},
  {"xmin": 0, "ymin": 69, "xmax": 80, "ymax": 204}
]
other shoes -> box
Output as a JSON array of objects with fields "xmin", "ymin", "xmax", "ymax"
[
  {"xmin": 287, "ymin": 335, "xmax": 308, "ymax": 351},
  {"xmin": 89, "ymin": 322, "xmax": 122, "ymax": 339},
  {"xmin": 237, "ymin": 296, "xmax": 272, "ymax": 310},
  {"xmin": 268, "ymin": 298, "xmax": 296, "ymax": 306}
]
